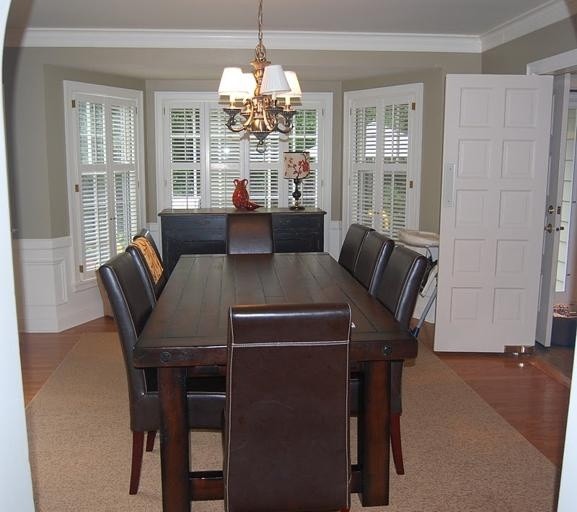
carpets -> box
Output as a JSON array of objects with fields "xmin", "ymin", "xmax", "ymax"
[{"xmin": 25, "ymin": 332, "xmax": 559, "ymax": 512}]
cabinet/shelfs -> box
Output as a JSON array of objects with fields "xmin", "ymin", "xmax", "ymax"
[{"xmin": 158, "ymin": 207, "xmax": 327, "ymax": 282}]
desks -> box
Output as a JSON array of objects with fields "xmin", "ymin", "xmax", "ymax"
[{"xmin": 133, "ymin": 252, "xmax": 420, "ymax": 508}]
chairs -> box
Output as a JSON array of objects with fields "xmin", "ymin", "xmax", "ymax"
[
  {"xmin": 227, "ymin": 213, "xmax": 271, "ymax": 256},
  {"xmin": 222, "ymin": 303, "xmax": 353, "ymax": 512},
  {"xmin": 355, "ymin": 232, "xmax": 393, "ymax": 292},
  {"xmin": 337, "ymin": 223, "xmax": 374, "ymax": 267},
  {"xmin": 129, "ymin": 233, "xmax": 222, "ymax": 376},
  {"xmin": 351, "ymin": 249, "xmax": 428, "ymax": 477},
  {"xmin": 94, "ymin": 253, "xmax": 227, "ymax": 497}
]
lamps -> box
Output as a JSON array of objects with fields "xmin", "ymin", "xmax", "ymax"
[
  {"xmin": 284, "ymin": 151, "xmax": 310, "ymax": 211},
  {"xmin": 217, "ymin": 0, "xmax": 302, "ymax": 153}
]
